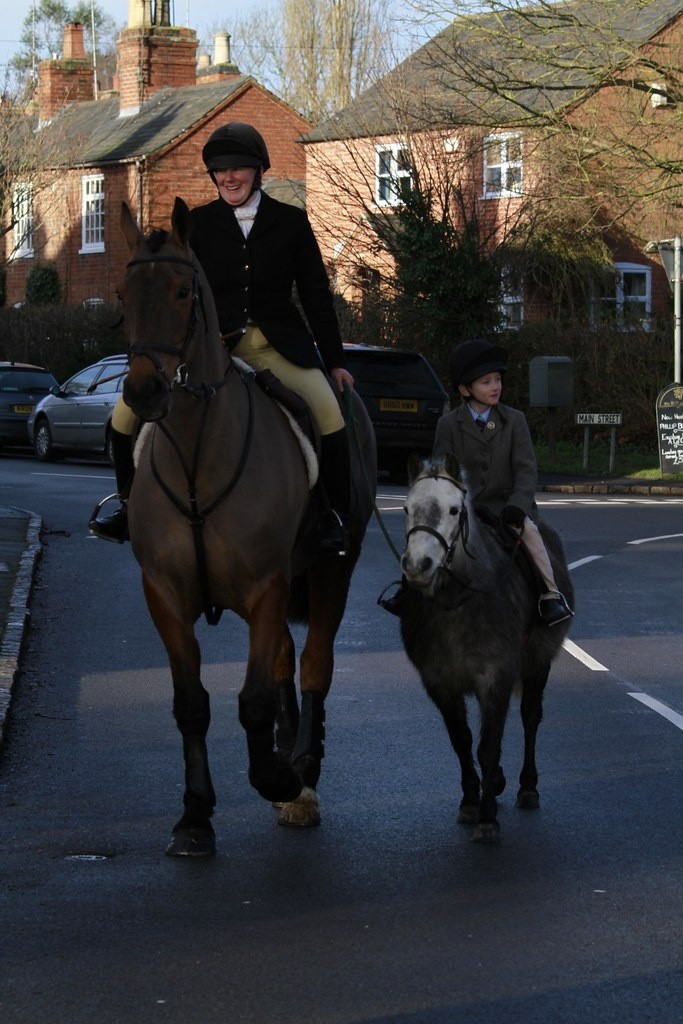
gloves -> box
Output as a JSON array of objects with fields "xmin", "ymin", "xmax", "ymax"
[{"xmin": 499, "ymin": 506, "xmax": 526, "ymax": 529}]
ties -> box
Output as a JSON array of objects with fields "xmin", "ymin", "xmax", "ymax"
[{"xmin": 475, "ymin": 414, "xmax": 486, "ymax": 434}]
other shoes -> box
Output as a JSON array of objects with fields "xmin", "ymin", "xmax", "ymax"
[
  {"xmin": 538, "ymin": 599, "xmax": 571, "ymax": 627},
  {"xmin": 383, "ymin": 589, "xmax": 404, "ymax": 618}
]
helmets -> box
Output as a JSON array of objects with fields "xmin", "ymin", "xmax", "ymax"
[
  {"xmin": 202, "ymin": 121, "xmax": 271, "ymax": 171},
  {"xmin": 447, "ymin": 340, "xmax": 510, "ymax": 394}
]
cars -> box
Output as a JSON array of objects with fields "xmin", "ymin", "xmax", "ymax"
[{"xmin": 0, "ymin": 360, "xmax": 61, "ymax": 451}]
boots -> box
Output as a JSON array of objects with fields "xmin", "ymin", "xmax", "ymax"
[
  {"xmin": 319, "ymin": 425, "xmax": 351, "ymax": 558},
  {"xmin": 89, "ymin": 426, "xmax": 138, "ymax": 541}
]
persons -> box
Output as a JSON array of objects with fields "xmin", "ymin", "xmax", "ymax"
[
  {"xmin": 89, "ymin": 123, "xmax": 351, "ymax": 550},
  {"xmin": 386, "ymin": 340, "xmax": 567, "ymax": 619}
]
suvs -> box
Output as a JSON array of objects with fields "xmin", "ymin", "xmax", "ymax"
[
  {"xmin": 312, "ymin": 340, "xmax": 450, "ymax": 490},
  {"xmin": 26, "ymin": 353, "xmax": 130, "ymax": 463}
]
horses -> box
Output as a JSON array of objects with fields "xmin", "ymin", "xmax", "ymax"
[
  {"xmin": 398, "ymin": 443, "xmax": 578, "ymax": 850},
  {"xmin": 115, "ymin": 197, "xmax": 376, "ymax": 860}
]
